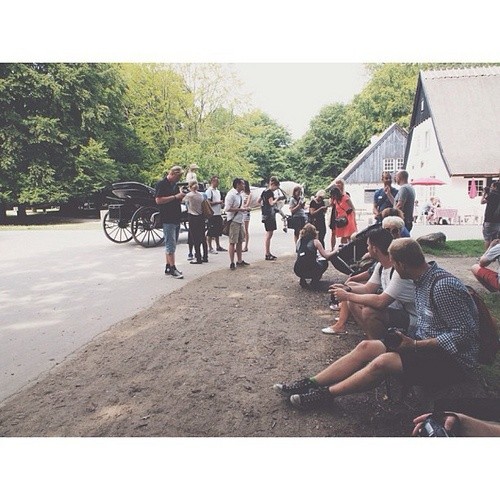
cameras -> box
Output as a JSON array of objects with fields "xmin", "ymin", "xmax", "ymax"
[
  {"xmin": 387, "ymin": 326, "xmax": 407, "ymax": 347},
  {"xmin": 420, "ymin": 416, "xmax": 455, "ymax": 437}
]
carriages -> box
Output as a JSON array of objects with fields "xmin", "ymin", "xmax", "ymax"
[{"xmin": 101, "ymin": 177, "xmax": 307, "ymax": 248}]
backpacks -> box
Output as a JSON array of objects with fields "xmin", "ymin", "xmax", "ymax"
[{"xmin": 428, "ymin": 273, "xmax": 498, "ymax": 365}]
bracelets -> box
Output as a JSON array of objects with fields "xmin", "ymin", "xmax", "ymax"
[
  {"xmin": 413, "ymin": 412, "xmax": 500, "ymax": 437},
  {"xmin": 415, "ymin": 339, "xmax": 417, "ymax": 351},
  {"xmin": 341, "ymin": 282, "xmax": 352, "ymax": 293},
  {"xmin": 174, "ymin": 195, "xmax": 177, "ymax": 201}
]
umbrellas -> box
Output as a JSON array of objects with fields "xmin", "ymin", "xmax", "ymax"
[
  {"xmin": 470, "ymin": 176, "xmax": 477, "ymax": 215},
  {"xmin": 410, "ymin": 178, "xmax": 447, "ymax": 201}
]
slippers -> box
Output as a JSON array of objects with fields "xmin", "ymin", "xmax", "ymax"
[
  {"xmin": 322, "ymin": 326, "xmax": 348, "ymax": 335},
  {"xmin": 334, "ymin": 316, "xmax": 357, "ymax": 325}
]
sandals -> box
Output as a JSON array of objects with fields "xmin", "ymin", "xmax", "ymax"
[{"xmin": 265, "ymin": 253, "xmax": 277, "ymax": 260}]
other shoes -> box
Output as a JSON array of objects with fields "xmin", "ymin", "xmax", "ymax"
[
  {"xmin": 244, "ymin": 247, "xmax": 247, "ymax": 251},
  {"xmin": 209, "ymin": 247, "xmax": 218, "ymax": 254},
  {"xmin": 216, "ymin": 247, "xmax": 227, "ymax": 251},
  {"xmin": 330, "ymin": 304, "xmax": 341, "ymax": 311},
  {"xmin": 299, "ymin": 278, "xmax": 320, "ymax": 289},
  {"xmin": 236, "ymin": 260, "xmax": 250, "ymax": 266},
  {"xmin": 189, "ymin": 258, "xmax": 208, "ymax": 264},
  {"xmin": 230, "ymin": 263, "xmax": 235, "ymax": 270},
  {"xmin": 188, "ymin": 254, "xmax": 193, "ymax": 260}
]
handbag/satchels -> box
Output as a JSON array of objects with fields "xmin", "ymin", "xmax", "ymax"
[
  {"xmin": 201, "ymin": 193, "xmax": 214, "ymax": 219},
  {"xmin": 334, "ymin": 216, "xmax": 348, "ymax": 227},
  {"xmin": 287, "ymin": 216, "xmax": 305, "ymax": 228},
  {"xmin": 221, "ymin": 218, "xmax": 233, "ymax": 236}
]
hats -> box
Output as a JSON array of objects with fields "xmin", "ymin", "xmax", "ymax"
[{"xmin": 189, "ymin": 164, "xmax": 200, "ymax": 169}]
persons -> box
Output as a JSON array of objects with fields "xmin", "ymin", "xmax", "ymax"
[
  {"xmin": 288, "ymin": 186, "xmax": 307, "ymax": 253},
  {"xmin": 155, "ymin": 166, "xmax": 186, "ymax": 279},
  {"xmin": 235, "ymin": 180, "xmax": 251, "ymax": 252},
  {"xmin": 373, "ymin": 170, "xmax": 398, "ymax": 224},
  {"xmin": 394, "ymin": 170, "xmax": 416, "ymax": 232},
  {"xmin": 182, "ymin": 181, "xmax": 208, "ymax": 264},
  {"xmin": 273, "ymin": 236, "xmax": 480, "ymax": 409},
  {"xmin": 329, "ymin": 179, "xmax": 358, "ymax": 252},
  {"xmin": 471, "ymin": 230, "xmax": 500, "ymax": 295},
  {"xmin": 205, "ymin": 176, "xmax": 228, "ymax": 254},
  {"xmin": 310, "ymin": 190, "xmax": 328, "ymax": 250},
  {"xmin": 185, "ymin": 164, "xmax": 200, "ymax": 183},
  {"xmin": 321, "ymin": 207, "xmax": 417, "ymax": 340},
  {"xmin": 258, "ymin": 176, "xmax": 287, "ymax": 260},
  {"xmin": 293, "ymin": 222, "xmax": 339, "ymax": 290},
  {"xmin": 481, "ymin": 181, "xmax": 500, "ymax": 250},
  {"xmin": 412, "ymin": 198, "xmax": 442, "ymax": 225},
  {"xmin": 224, "ymin": 178, "xmax": 253, "ymax": 270}
]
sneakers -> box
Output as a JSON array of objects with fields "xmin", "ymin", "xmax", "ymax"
[
  {"xmin": 170, "ymin": 265, "xmax": 183, "ymax": 278},
  {"xmin": 289, "ymin": 386, "xmax": 334, "ymax": 410},
  {"xmin": 273, "ymin": 378, "xmax": 318, "ymax": 399},
  {"xmin": 165, "ymin": 264, "xmax": 182, "ymax": 275}
]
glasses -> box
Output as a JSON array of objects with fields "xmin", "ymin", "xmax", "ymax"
[{"xmin": 386, "ymin": 227, "xmax": 401, "ymax": 235}]
